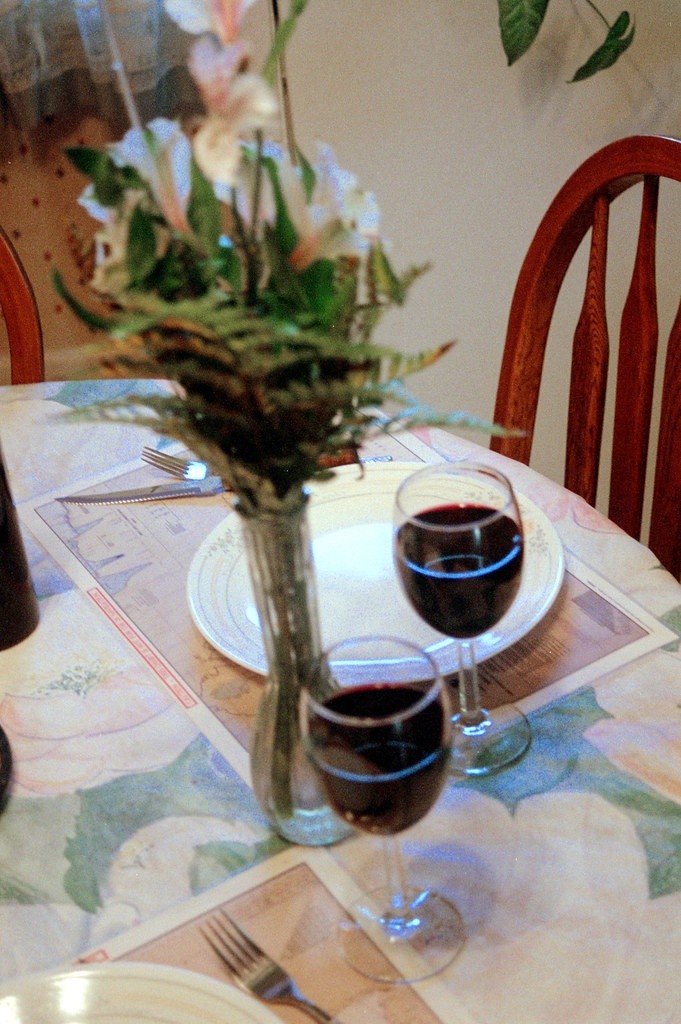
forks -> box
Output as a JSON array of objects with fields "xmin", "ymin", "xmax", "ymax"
[
  {"xmin": 194, "ymin": 907, "xmax": 336, "ymax": 1024},
  {"xmin": 140, "ymin": 446, "xmax": 208, "ymax": 480}
]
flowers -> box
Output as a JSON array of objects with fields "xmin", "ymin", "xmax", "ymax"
[{"xmin": 52, "ymin": 0, "xmax": 636, "ymax": 820}]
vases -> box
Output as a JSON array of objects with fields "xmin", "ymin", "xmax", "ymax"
[{"xmin": 233, "ymin": 489, "xmax": 357, "ymax": 848}]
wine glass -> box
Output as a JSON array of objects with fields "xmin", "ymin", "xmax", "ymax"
[
  {"xmin": 299, "ymin": 634, "xmax": 467, "ymax": 984},
  {"xmin": 391, "ymin": 462, "xmax": 533, "ymax": 777}
]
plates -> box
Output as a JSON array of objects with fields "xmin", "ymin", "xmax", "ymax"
[
  {"xmin": 186, "ymin": 461, "xmax": 564, "ymax": 677},
  {"xmin": 0, "ymin": 961, "xmax": 284, "ymax": 1024}
]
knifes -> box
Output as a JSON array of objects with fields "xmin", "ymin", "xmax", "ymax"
[{"xmin": 54, "ymin": 446, "xmax": 358, "ymax": 506}]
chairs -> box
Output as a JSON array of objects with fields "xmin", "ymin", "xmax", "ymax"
[{"xmin": 492, "ymin": 133, "xmax": 681, "ymax": 584}]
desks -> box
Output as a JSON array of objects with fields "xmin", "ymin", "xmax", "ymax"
[{"xmin": 0, "ymin": 379, "xmax": 681, "ymax": 1024}]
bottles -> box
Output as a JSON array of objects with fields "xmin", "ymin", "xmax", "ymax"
[{"xmin": 0, "ymin": 444, "xmax": 40, "ymax": 653}]
